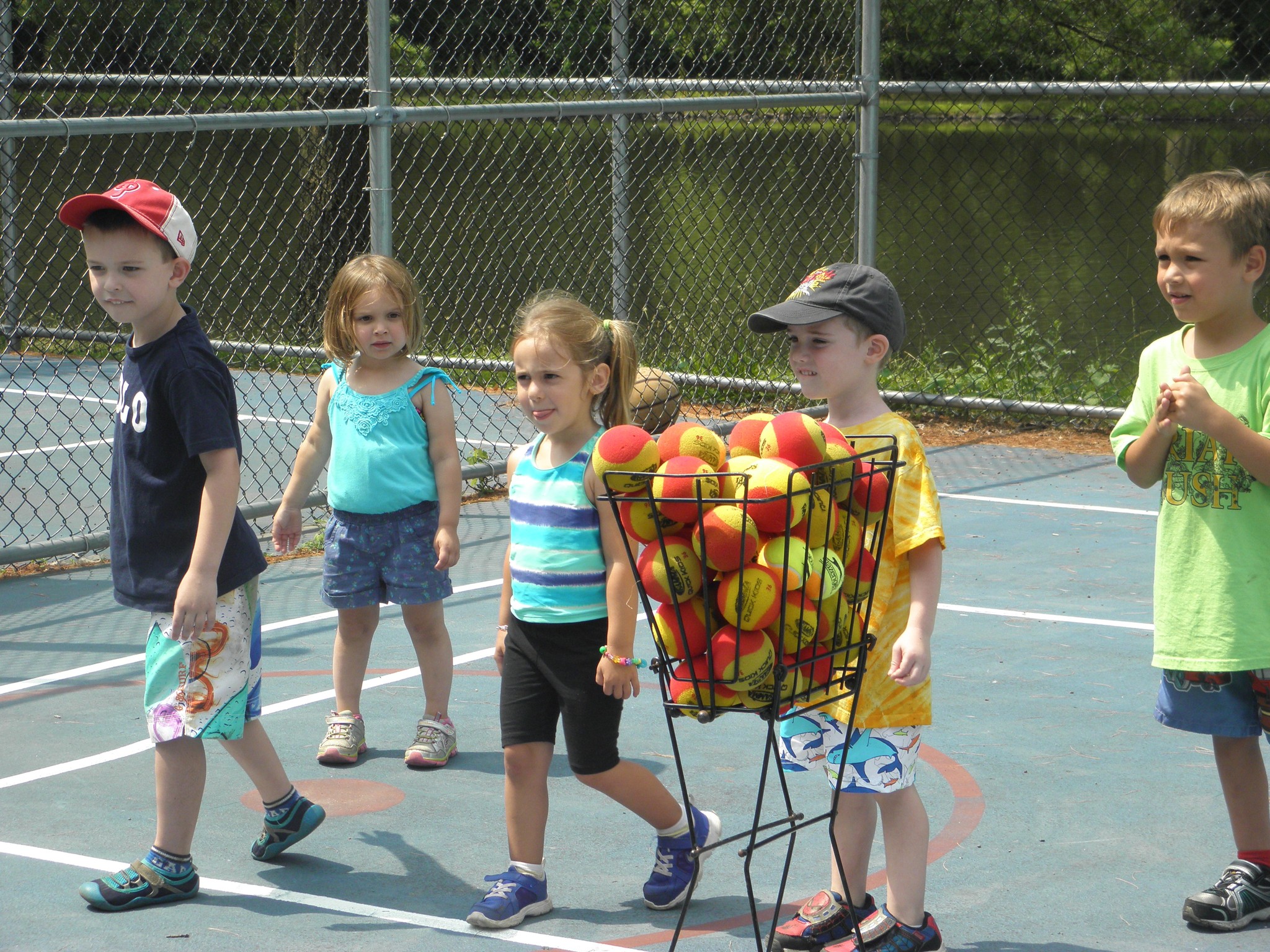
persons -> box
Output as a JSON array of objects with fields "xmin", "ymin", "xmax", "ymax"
[
  {"xmin": 748, "ymin": 261, "xmax": 946, "ymax": 952},
  {"xmin": 1109, "ymin": 169, "xmax": 1270, "ymax": 932},
  {"xmin": 270, "ymin": 253, "xmax": 465, "ymax": 770},
  {"xmin": 59, "ymin": 179, "xmax": 326, "ymax": 911},
  {"xmin": 464, "ymin": 289, "xmax": 722, "ymax": 927}
]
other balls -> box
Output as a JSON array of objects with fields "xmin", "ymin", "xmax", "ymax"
[{"xmin": 591, "ymin": 411, "xmax": 891, "ymax": 724}]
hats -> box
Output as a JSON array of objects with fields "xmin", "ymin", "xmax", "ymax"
[
  {"xmin": 59, "ymin": 179, "xmax": 198, "ymax": 266},
  {"xmin": 747, "ymin": 262, "xmax": 905, "ymax": 352}
]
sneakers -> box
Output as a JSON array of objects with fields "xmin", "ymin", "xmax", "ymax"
[
  {"xmin": 250, "ymin": 796, "xmax": 326, "ymax": 861},
  {"xmin": 316, "ymin": 710, "xmax": 367, "ymax": 764},
  {"xmin": 821, "ymin": 903, "xmax": 946, "ymax": 952},
  {"xmin": 1182, "ymin": 859, "xmax": 1270, "ymax": 932},
  {"xmin": 764, "ymin": 888, "xmax": 878, "ymax": 952},
  {"xmin": 466, "ymin": 857, "xmax": 553, "ymax": 929},
  {"xmin": 79, "ymin": 859, "xmax": 199, "ymax": 911},
  {"xmin": 404, "ymin": 716, "xmax": 458, "ymax": 767},
  {"xmin": 642, "ymin": 803, "xmax": 722, "ymax": 910}
]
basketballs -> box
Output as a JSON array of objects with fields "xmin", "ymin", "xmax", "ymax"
[{"xmin": 628, "ymin": 366, "xmax": 682, "ymax": 436}]
